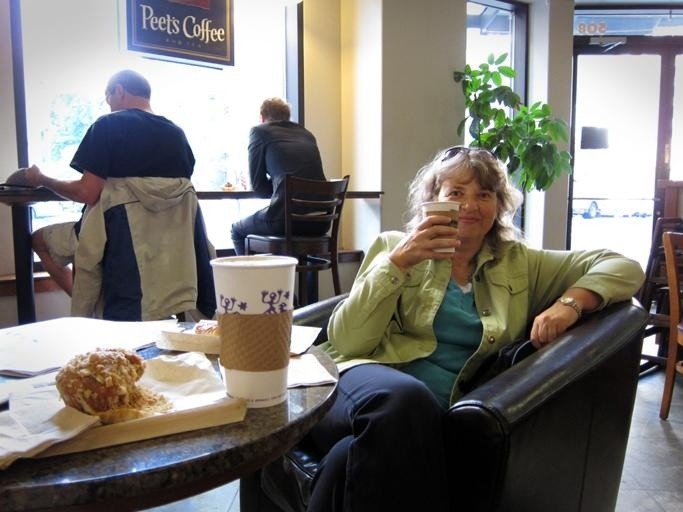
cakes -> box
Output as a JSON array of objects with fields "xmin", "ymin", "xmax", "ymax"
[
  {"xmin": 195, "ymin": 322, "xmax": 219, "ymax": 335},
  {"xmin": 55, "ymin": 348, "xmax": 146, "ymax": 425}
]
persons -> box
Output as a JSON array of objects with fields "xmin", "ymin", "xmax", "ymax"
[
  {"xmin": 24, "ymin": 68, "xmax": 196, "ymax": 303},
  {"xmin": 305, "ymin": 144, "xmax": 647, "ymax": 512},
  {"xmin": 229, "ymin": 97, "xmax": 331, "ymax": 260}
]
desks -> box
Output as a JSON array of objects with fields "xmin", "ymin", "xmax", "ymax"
[{"xmin": 1, "ymin": 180, "xmax": 387, "ymax": 331}]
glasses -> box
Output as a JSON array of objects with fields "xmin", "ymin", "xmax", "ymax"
[{"xmin": 442, "ymin": 147, "xmax": 498, "ymax": 167}]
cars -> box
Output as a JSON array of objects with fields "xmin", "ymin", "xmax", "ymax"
[{"xmin": 572, "ymin": 199, "xmax": 656, "ymax": 218}]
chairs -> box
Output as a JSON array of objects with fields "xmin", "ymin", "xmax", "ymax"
[
  {"xmin": 241, "ymin": 169, "xmax": 351, "ymax": 303},
  {"xmin": 234, "ymin": 290, "xmax": 647, "ymax": 510},
  {"xmin": 654, "ymin": 230, "xmax": 683, "ymax": 421}
]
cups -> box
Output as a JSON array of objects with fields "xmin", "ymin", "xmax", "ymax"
[
  {"xmin": 421, "ymin": 200, "xmax": 463, "ymax": 253},
  {"xmin": 210, "ymin": 255, "xmax": 299, "ymax": 409}
]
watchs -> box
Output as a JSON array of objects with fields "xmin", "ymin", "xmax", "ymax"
[{"xmin": 556, "ymin": 295, "xmax": 583, "ymax": 318}]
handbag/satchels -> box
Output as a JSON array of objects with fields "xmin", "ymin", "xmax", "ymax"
[{"xmin": 458, "ymin": 338, "xmax": 533, "ymax": 399}]
